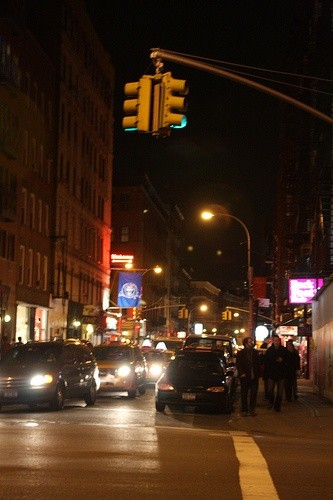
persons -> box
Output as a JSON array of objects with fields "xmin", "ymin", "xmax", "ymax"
[
  {"xmin": 263, "ymin": 335, "xmax": 300, "ymax": 412},
  {"xmin": 0, "ymin": 336, "xmax": 11, "ymax": 354},
  {"xmin": 235, "ymin": 337, "xmax": 261, "ymax": 415},
  {"xmin": 14, "ymin": 337, "xmax": 23, "ymax": 346}
]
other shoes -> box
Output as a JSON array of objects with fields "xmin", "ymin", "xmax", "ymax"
[
  {"xmin": 285, "ymin": 394, "xmax": 299, "ymax": 402},
  {"xmin": 241, "ymin": 410, "xmax": 248, "ymax": 417},
  {"xmin": 273, "ymin": 406, "xmax": 280, "ymax": 412},
  {"xmin": 267, "ymin": 403, "xmax": 272, "ymax": 409},
  {"xmin": 248, "ymin": 409, "xmax": 258, "ymax": 416}
]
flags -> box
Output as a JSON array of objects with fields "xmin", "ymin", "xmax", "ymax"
[{"xmin": 119, "ymin": 272, "xmax": 142, "ymax": 308}]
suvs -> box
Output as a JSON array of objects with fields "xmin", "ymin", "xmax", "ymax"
[
  {"xmin": 91, "ymin": 345, "xmax": 147, "ymax": 399},
  {"xmin": 0, "ymin": 338, "xmax": 103, "ymax": 413}
]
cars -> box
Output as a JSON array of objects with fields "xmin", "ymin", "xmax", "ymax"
[
  {"xmin": 155, "ymin": 351, "xmax": 239, "ymax": 413},
  {"xmin": 141, "ymin": 335, "xmax": 240, "ymax": 387}
]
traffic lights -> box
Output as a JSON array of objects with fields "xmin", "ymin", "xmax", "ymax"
[
  {"xmin": 151, "ymin": 72, "xmax": 189, "ymax": 137},
  {"xmin": 119, "ymin": 73, "xmax": 154, "ymax": 132}
]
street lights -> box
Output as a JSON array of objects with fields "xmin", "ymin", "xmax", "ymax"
[
  {"xmin": 136, "ymin": 265, "xmax": 162, "ymax": 344},
  {"xmin": 201, "ymin": 209, "xmax": 255, "ymax": 341}
]
